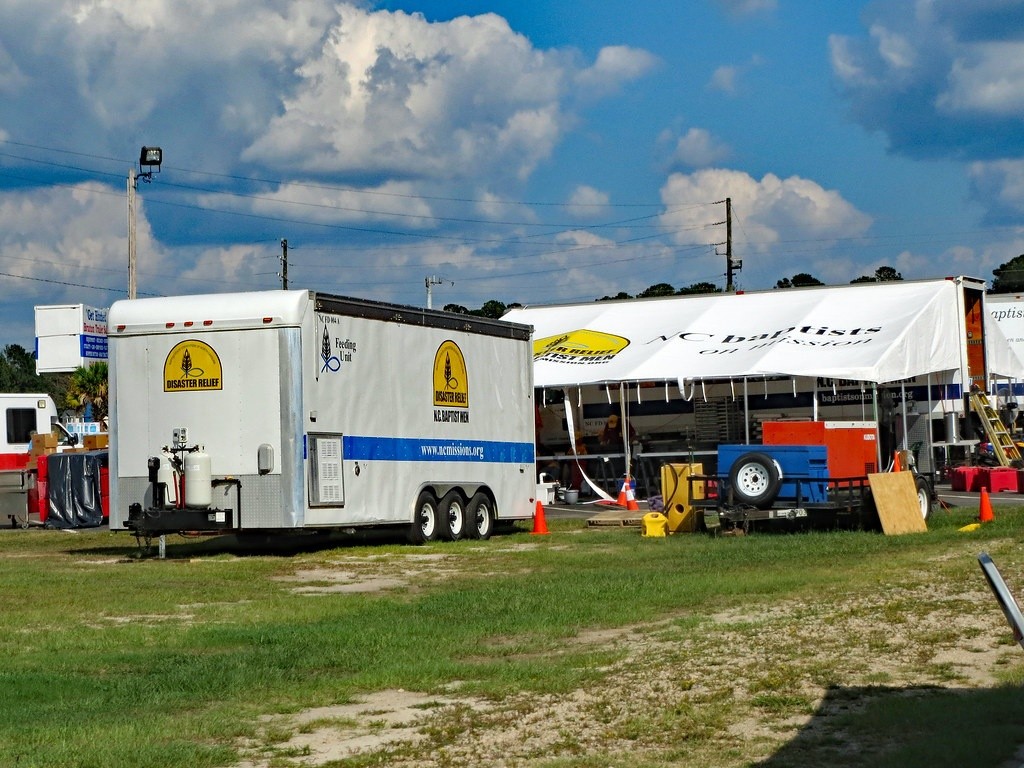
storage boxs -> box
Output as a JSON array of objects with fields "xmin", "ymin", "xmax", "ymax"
[
  {"xmin": 63, "ymin": 433, "xmax": 109, "ymax": 453},
  {"xmin": 27, "ymin": 433, "xmax": 58, "ymax": 468}
]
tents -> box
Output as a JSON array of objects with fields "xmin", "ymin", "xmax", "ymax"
[{"xmin": 497, "ymin": 280, "xmax": 968, "ymax": 488}]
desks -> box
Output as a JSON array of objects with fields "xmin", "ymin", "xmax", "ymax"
[{"xmin": 930, "ymin": 439, "xmax": 981, "ymax": 465}]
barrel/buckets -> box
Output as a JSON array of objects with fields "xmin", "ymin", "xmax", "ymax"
[{"xmin": 562, "ymin": 484, "xmax": 578, "ymax": 504}]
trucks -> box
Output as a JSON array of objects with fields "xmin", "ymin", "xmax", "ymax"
[{"xmin": 0, "ymin": 392, "xmax": 84, "ymax": 453}]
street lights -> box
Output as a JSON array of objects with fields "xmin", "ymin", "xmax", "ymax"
[{"xmin": 127, "ymin": 145, "xmax": 163, "ymax": 300}]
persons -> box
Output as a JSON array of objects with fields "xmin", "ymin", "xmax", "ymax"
[
  {"xmin": 595, "ymin": 416, "xmax": 637, "ymax": 478},
  {"xmin": 567, "ymin": 431, "xmax": 588, "ymax": 491}
]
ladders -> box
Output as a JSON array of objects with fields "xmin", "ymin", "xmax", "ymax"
[{"xmin": 970, "ymin": 390, "xmax": 1023, "ymax": 468}]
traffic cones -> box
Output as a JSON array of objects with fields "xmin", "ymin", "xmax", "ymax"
[
  {"xmin": 624, "ymin": 484, "xmax": 639, "ymax": 510},
  {"xmin": 529, "ymin": 501, "xmax": 551, "ymax": 535},
  {"xmin": 615, "ymin": 476, "xmax": 630, "ymax": 507},
  {"xmin": 978, "ymin": 486, "xmax": 993, "ymax": 522},
  {"xmin": 893, "ymin": 450, "xmax": 900, "ymax": 473}
]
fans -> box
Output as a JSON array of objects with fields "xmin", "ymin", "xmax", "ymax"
[{"xmin": 996, "ymin": 389, "xmax": 1019, "ymax": 435}]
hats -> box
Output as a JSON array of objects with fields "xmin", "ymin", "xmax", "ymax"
[
  {"xmin": 575, "ymin": 431, "xmax": 583, "ymax": 439},
  {"xmin": 608, "ymin": 415, "xmax": 618, "ymax": 428}
]
